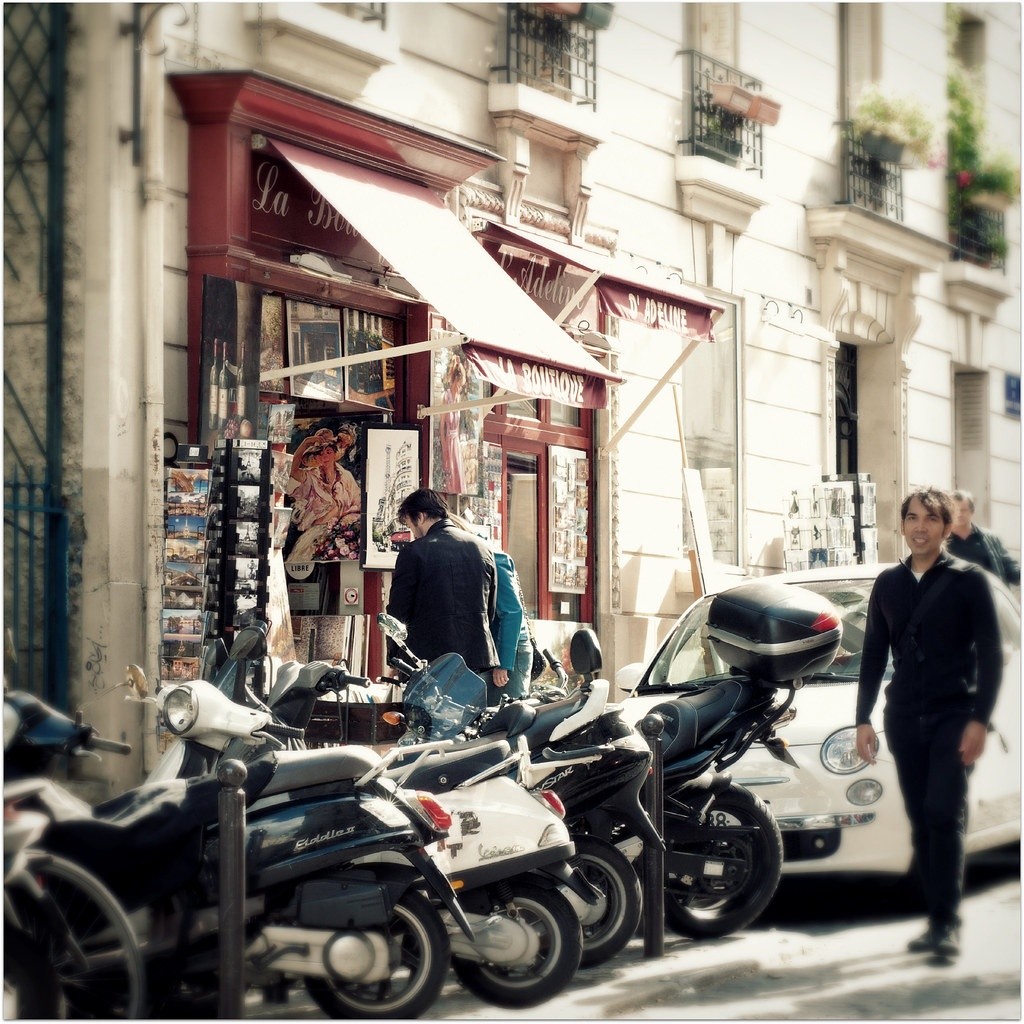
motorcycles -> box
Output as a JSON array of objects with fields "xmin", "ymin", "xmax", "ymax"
[{"xmin": 4, "ymin": 582, "xmax": 844, "ymax": 1019}]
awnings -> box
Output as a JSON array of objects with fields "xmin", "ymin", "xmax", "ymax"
[
  {"xmin": 477, "ymin": 217, "xmax": 725, "ymax": 449},
  {"xmin": 261, "ymin": 136, "xmax": 627, "ymax": 414}
]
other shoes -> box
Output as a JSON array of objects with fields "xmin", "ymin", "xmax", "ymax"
[
  {"xmin": 933, "ymin": 919, "xmax": 961, "ymax": 958},
  {"xmin": 907, "ymin": 919, "xmax": 936, "ymax": 952}
]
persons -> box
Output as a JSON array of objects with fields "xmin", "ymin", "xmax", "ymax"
[
  {"xmin": 945, "ymin": 490, "xmax": 1020, "ymax": 590},
  {"xmin": 386, "ymin": 489, "xmax": 499, "ymax": 708},
  {"xmin": 450, "ymin": 511, "xmax": 532, "ymax": 708},
  {"xmin": 855, "ymin": 486, "xmax": 1004, "ymax": 958}
]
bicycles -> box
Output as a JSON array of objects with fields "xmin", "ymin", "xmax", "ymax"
[{"xmin": 3, "ymin": 777, "xmax": 149, "ymax": 1018}]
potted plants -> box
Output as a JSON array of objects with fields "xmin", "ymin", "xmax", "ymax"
[
  {"xmin": 962, "ymin": 161, "xmax": 1016, "ymax": 210},
  {"xmin": 850, "ymin": 95, "xmax": 938, "ymax": 165}
]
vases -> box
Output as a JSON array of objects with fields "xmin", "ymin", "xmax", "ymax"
[
  {"xmin": 708, "ymin": 78, "xmax": 782, "ymax": 126},
  {"xmin": 533, "ymin": 2, "xmax": 615, "ymax": 32}
]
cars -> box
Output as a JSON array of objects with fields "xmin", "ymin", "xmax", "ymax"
[{"xmin": 608, "ymin": 563, "xmax": 1021, "ymax": 914}]
